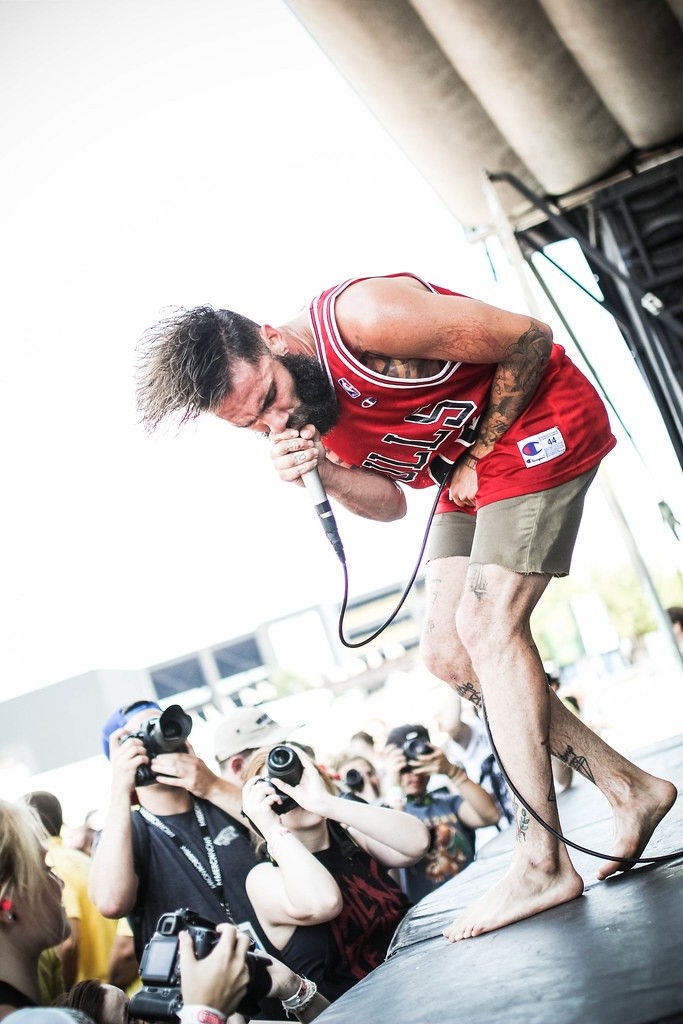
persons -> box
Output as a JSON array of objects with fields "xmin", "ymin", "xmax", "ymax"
[
  {"xmin": 0, "ymin": 671, "xmax": 581, "ymax": 1024},
  {"xmin": 666, "ymin": 606, "xmax": 683, "ymax": 654},
  {"xmin": 136, "ymin": 271, "xmax": 679, "ymax": 943}
]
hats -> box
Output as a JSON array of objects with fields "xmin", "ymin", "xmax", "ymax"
[
  {"xmin": 213, "ymin": 707, "xmax": 305, "ymax": 764},
  {"xmin": 388, "ymin": 723, "xmax": 429, "ymax": 750},
  {"xmin": 103, "ymin": 699, "xmax": 163, "ymax": 762}
]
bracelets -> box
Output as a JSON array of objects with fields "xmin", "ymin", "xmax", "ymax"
[
  {"xmin": 267, "ymin": 829, "xmax": 291, "ymax": 854},
  {"xmin": 449, "ymin": 765, "xmax": 468, "ymax": 785},
  {"xmin": 281, "ymin": 975, "xmax": 317, "ymax": 1018},
  {"xmin": 181, "ymin": 1004, "xmax": 225, "ymax": 1024}
]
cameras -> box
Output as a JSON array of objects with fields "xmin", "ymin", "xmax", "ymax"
[
  {"xmin": 344, "ymin": 770, "xmax": 363, "ymax": 793},
  {"xmin": 118, "ymin": 704, "xmax": 192, "ymax": 787},
  {"xmin": 398, "ymin": 740, "xmax": 433, "ymax": 774},
  {"xmin": 254, "ymin": 746, "xmax": 304, "ymax": 815},
  {"xmin": 127, "ymin": 906, "xmax": 274, "ymax": 1024}
]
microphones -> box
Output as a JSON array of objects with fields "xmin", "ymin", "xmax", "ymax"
[{"xmin": 300, "ymin": 467, "xmax": 346, "ymax": 562}]
